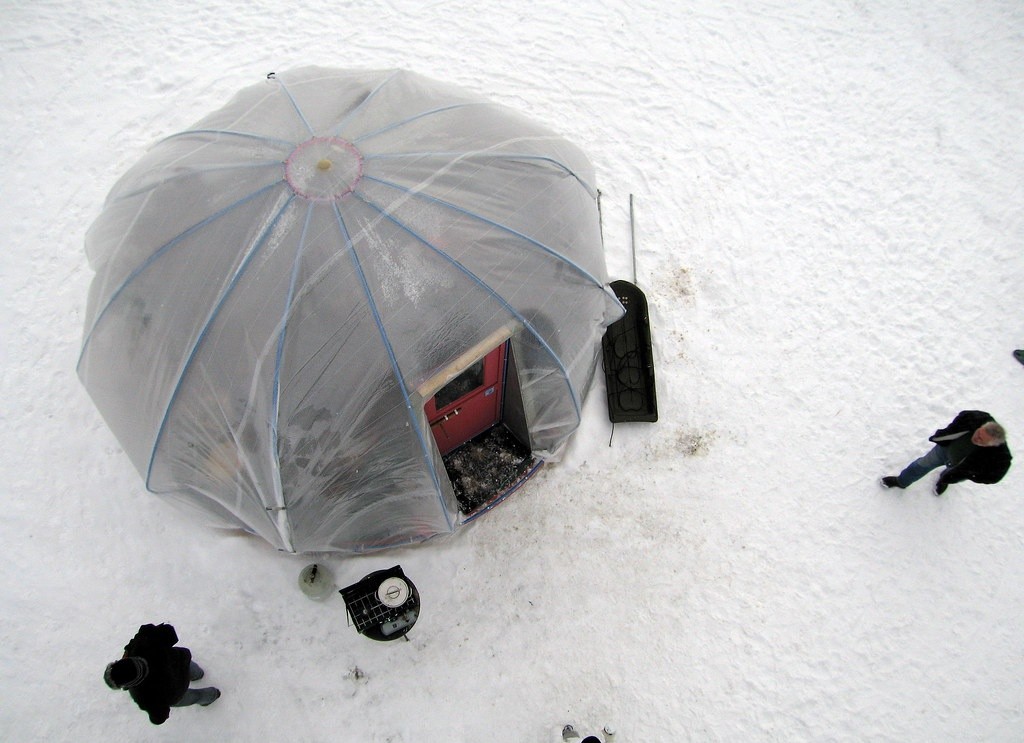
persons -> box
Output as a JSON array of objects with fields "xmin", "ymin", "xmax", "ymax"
[
  {"xmin": 103, "ymin": 623, "xmax": 221, "ymax": 725},
  {"xmin": 881, "ymin": 411, "xmax": 1012, "ymax": 497}
]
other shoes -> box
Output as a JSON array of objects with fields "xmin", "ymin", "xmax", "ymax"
[
  {"xmin": 934, "ymin": 483, "xmax": 948, "ymax": 495},
  {"xmin": 881, "ymin": 476, "xmax": 906, "ymax": 489}
]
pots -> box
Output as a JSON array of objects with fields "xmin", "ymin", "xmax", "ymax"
[{"xmin": 374, "ymin": 577, "xmax": 412, "ymax": 609}]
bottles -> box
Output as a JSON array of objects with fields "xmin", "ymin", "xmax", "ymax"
[{"xmin": 298, "ymin": 563, "xmax": 333, "ymax": 602}]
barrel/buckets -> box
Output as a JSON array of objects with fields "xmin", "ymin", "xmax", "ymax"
[{"xmin": 602, "ymin": 723, "xmax": 615, "ymax": 736}]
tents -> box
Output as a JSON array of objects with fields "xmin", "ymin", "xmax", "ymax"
[{"xmin": 75, "ymin": 65, "xmax": 624, "ymax": 555}]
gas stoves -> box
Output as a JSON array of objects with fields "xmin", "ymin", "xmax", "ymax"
[{"xmin": 339, "ymin": 564, "xmax": 420, "ymax": 635}]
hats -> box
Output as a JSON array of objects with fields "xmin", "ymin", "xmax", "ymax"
[{"xmin": 104, "ymin": 657, "xmax": 144, "ymax": 690}]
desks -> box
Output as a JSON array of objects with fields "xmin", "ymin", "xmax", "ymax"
[{"xmin": 345, "ymin": 569, "xmax": 420, "ymax": 641}]
